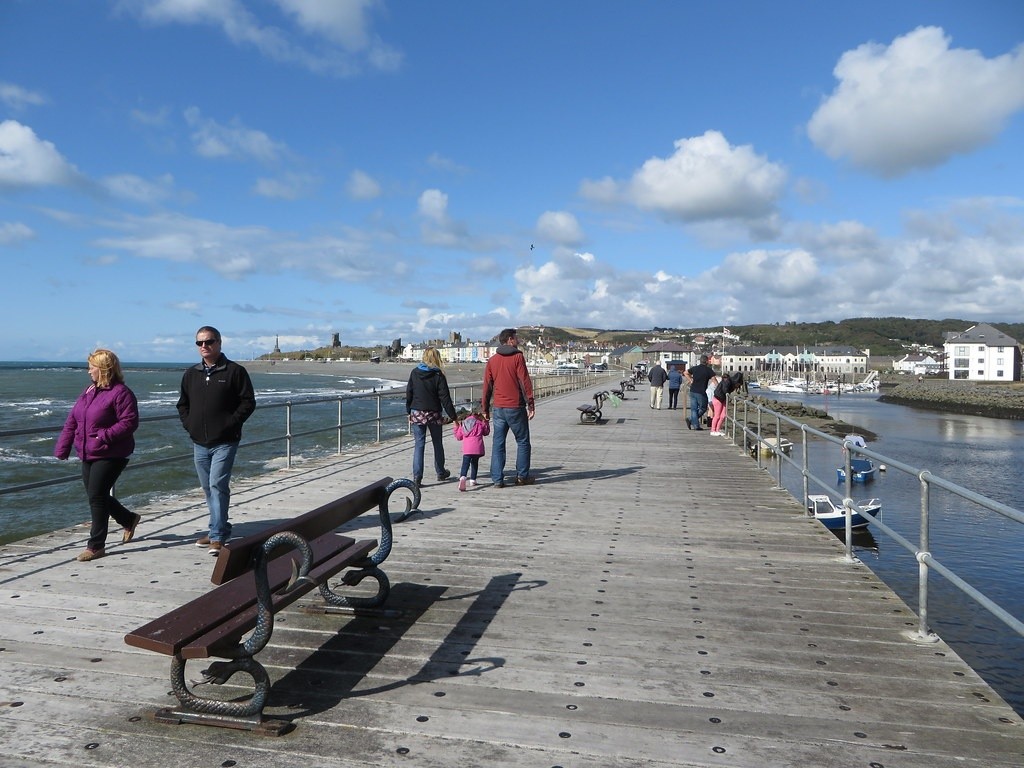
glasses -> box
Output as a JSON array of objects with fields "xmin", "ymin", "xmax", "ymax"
[{"xmin": 196, "ymin": 340, "xmax": 217, "ymax": 346}]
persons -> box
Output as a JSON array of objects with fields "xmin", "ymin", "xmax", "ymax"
[
  {"xmin": 54, "ymin": 350, "xmax": 141, "ymax": 561},
  {"xmin": 453, "ymin": 413, "xmax": 490, "ymax": 491},
  {"xmin": 176, "ymin": 326, "xmax": 256, "ymax": 554},
  {"xmin": 684, "ymin": 355, "xmax": 717, "ymax": 431},
  {"xmin": 406, "ymin": 347, "xmax": 459, "ymax": 488},
  {"xmin": 710, "ymin": 373, "xmax": 743, "ymax": 436},
  {"xmin": 705, "ymin": 374, "xmax": 730, "ymax": 427},
  {"xmin": 482, "ymin": 329, "xmax": 535, "ymax": 488},
  {"xmin": 666, "ymin": 365, "xmax": 682, "ymax": 409},
  {"xmin": 648, "ymin": 361, "xmax": 667, "ymax": 410}
]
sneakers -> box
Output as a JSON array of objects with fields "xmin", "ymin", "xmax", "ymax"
[
  {"xmin": 436, "ymin": 469, "xmax": 450, "ymax": 481},
  {"xmin": 414, "ymin": 478, "xmax": 422, "ymax": 488},
  {"xmin": 196, "ymin": 530, "xmax": 232, "ymax": 547},
  {"xmin": 208, "ymin": 540, "xmax": 225, "ymax": 556}
]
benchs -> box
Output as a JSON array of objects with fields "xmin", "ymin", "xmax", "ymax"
[
  {"xmin": 124, "ymin": 477, "xmax": 422, "ymax": 736},
  {"xmin": 610, "ymin": 370, "xmax": 645, "ymax": 399},
  {"xmin": 577, "ymin": 391, "xmax": 609, "ymax": 425}
]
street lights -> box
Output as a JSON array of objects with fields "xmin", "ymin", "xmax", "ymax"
[
  {"xmin": 349, "ymin": 350, "xmax": 352, "ymax": 362},
  {"xmin": 371, "ymin": 351, "xmax": 375, "ymax": 358},
  {"xmin": 391, "ymin": 349, "xmax": 393, "ymax": 359}
]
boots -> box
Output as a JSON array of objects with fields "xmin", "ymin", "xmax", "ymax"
[{"xmin": 707, "ymin": 417, "xmax": 712, "ymax": 427}]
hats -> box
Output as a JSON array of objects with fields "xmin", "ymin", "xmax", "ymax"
[{"xmin": 656, "ymin": 361, "xmax": 661, "ymax": 365}]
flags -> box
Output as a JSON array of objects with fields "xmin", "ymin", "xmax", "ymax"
[{"xmin": 723, "ymin": 327, "xmax": 730, "ymax": 336}]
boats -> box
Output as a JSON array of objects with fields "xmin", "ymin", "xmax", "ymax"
[
  {"xmin": 548, "ymin": 364, "xmax": 579, "ymax": 375},
  {"xmin": 805, "ymin": 494, "xmax": 884, "ymax": 531},
  {"xmin": 760, "ymin": 437, "xmax": 794, "ymax": 459},
  {"xmin": 844, "ymin": 432, "xmax": 868, "ymax": 456},
  {"xmin": 835, "ymin": 456, "xmax": 878, "ymax": 488},
  {"xmin": 748, "ymin": 344, "xmax": 882, "ymax": 394}
]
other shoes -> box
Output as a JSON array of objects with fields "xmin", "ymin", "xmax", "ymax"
[
  {"xmin": 695, "ymin": 427, "xmax": 703, "ymax": 430},
  {"xmin": 122, "ymin": 513, "xmax": 140, "ymax": 543},
  {"xmin": 457, "ymin": 476, "xmax": 466, "ymax": 491},
  {"xmin": 685, "ymin": 418, "xmax": 691, "ymax": 430},
  {"xmin": 494, "ymin": 483, "xmax": 504, "ymax": 488},
  {"xmin": 515, "ymin": 477, "xmax": 535, "ymax": 486},
  {"xmin": 710, "ymin": 432, "xmax": 725, "ymax": 436},
  {"xmin": 649, "ymin": 405, "xmax": 654, "ymax": 409},
  {"xmin": 76, "ymin": 547, "xmax": 105, "ymax": 562},
  {"xmin": 469, "ymin": 479, "xmax": 479, "ymax": 487}
]
